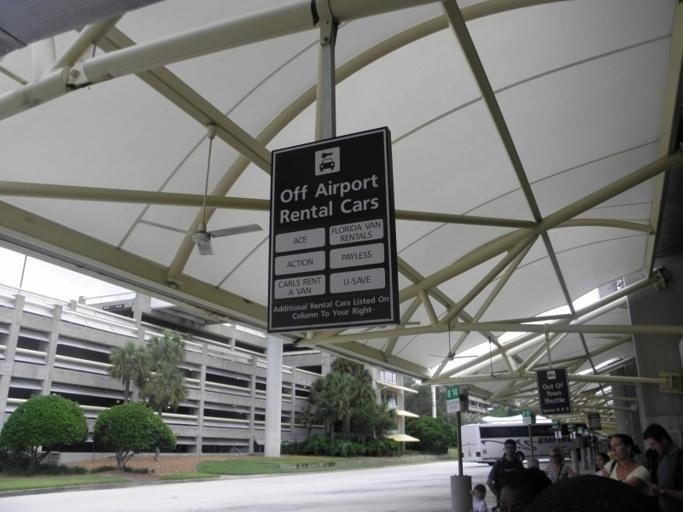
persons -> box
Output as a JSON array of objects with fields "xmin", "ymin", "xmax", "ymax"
[{"xmin": 470, "ymin": 422, "xmax": 683, "ymax": 512}]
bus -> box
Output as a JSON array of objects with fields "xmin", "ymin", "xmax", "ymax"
[{"xmin": 459, "ymin": 420, "xmax": 594, "ymax": 467}]
